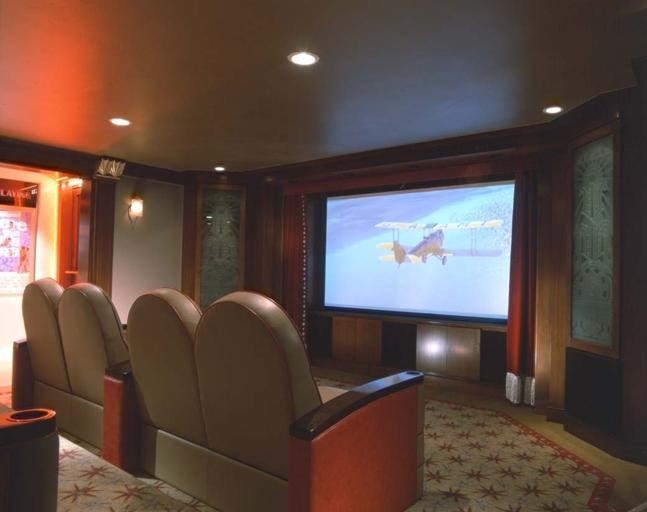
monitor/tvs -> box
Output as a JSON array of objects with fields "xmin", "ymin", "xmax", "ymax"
[{"xmin": 321, "ymin": 179, "xmax": 516, "ymax": 323}]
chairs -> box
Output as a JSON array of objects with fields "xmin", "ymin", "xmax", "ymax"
[
  {"xmin": 9, "ymin": 277, "xmax": 68, "ymax": 414},
  {"xmin": 191, "ymin": 290, "xmax": 428, "ymax": 510},
  {"xmin": 57, "ymin": 280, "xmax": 128, "ymax": 461},
  {"xmin": 10, "ymin": 406, "xmax": 60, "ymax": 510},
  {"xmin": 126, "ymin": 285, "xmax": 202, "ymax": 499}
]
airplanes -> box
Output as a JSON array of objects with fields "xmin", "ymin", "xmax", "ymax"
[{"xmin": 374, "ymin": 219, "xmax": 504, "ymax": 266}]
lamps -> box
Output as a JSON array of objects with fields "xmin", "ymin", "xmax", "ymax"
[{"xmin": 126, "ymin": 191, "xmax": 145, "ymax": 224}]
